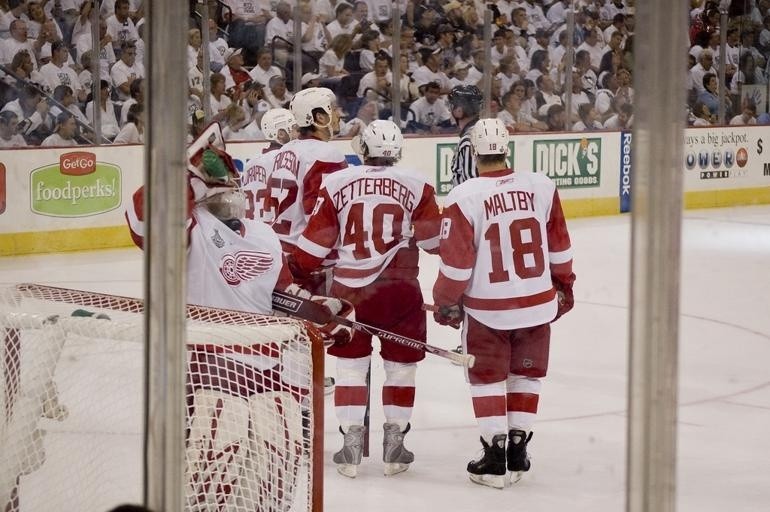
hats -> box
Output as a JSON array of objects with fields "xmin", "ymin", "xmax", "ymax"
[
  {"xmin": 454, "ymin": 61, "xmax": 472, "ymax": 73},
  {"xmin": 536, "ymin": 30, "xmax": 553, "ymax": 38},
  {"xmin": 244, "ymin": 75, "xmax": 286, "ymax": 91},
  {"xmin": 301, "ymin": 72, "xmax": 321, "ymax": 86},
  {"xmin": 437, "ymin": 24, "xmax": 457, "ymax": 33},
  {"xmin": 223, "ymin": 47, "xmax": 243, "ymax": 65}
]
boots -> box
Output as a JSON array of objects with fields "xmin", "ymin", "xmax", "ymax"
[
  {"xmin": 332, "ymin": 424, "xmax": 365, "ymax": 466},
  {"xmin": 507, "ymin": 429, "xmax": 530, "ymax": 471},
  {"xmin": 383, "ymin": 422, "xmax": 413, "ymax": 464},
  {"xmin": 467, "ymin": 433, "xmax": 506, "ymax": 475}
]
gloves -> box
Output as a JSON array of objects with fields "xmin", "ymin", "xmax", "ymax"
[
  {"xmin": 433, "ymin": 303, "xmax": 462, "ymax": 329},
  {"xmin": 189, "ymin": 122, "xmax": 245, "ymax": 202},
  {"xmin": 553, "ymin": 273, "xmax": 576, "ymax": 323},
  {"xmin": 297, "ymin": 289, "xmax": 356, "ymax": 347}
]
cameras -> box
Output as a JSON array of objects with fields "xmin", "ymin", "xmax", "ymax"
[{"xmin": 40, "ymin": 87, "xmax": 52, "ymax": 101}]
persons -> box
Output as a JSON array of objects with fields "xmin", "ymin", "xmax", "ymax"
[
  {"xmin": 241, "ymin": 104, "xmax": 294, "ymax": 224},
  {"xmin": 266, "ymin": 87, "xmax": 349, "ymax": 394},
  {"xmin": 445, "ymin": 84, "xmax": 485, "ymax": 184},
  {"xmin": 288, "ymin": 118, "xmax": 443, "ymax": 466},
  {"xmin": 123, "ymin": 141, "xmax": 351, "ymax": 512},
  {"xmin": 1, "ymin": 0, "xmax": 769, "ymax": 147},
  {"xmin": 430, "ymin": 120, "xmax": 575, "ymax": 472}
]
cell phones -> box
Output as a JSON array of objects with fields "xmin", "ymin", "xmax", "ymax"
[{"xmin": 257, "ymin": 95, "xmax": 262, "ymax": 100}]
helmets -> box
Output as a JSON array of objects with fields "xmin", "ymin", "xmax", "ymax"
[
  {"xmin": 359, "ymin": 119, "xmax": 403, "ymax": 158},
  {"xmin": 290, "ymin": 87, "xmax": 336, "ymax": 127},
  {"xmin": 260, "ymin": 108, "xmax": 298, "ymax": 146},
  {"xmin": 471, "ymin": 119, "xmax": 509, "ymax": 156},
  {"xmin": 448, "ymin": 85, "xmax": 483, "ymax": 117}
]
